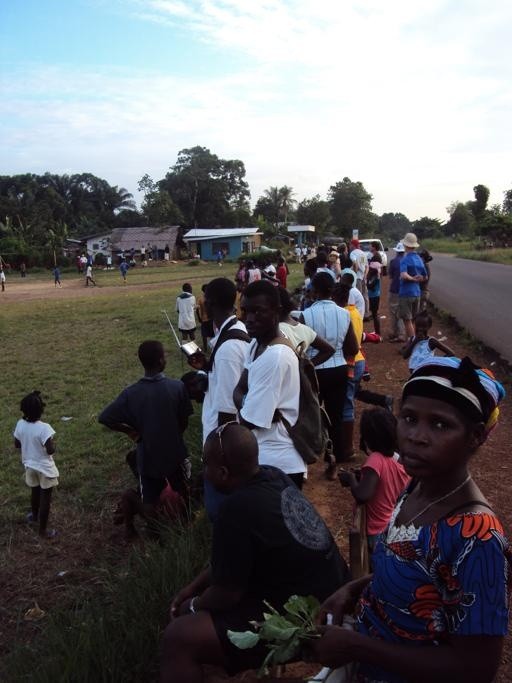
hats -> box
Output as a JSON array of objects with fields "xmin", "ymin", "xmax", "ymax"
[
  {"xmin": 392, "ymin": 242, "xmax": 405, "ymax": 252},
  {"xmin": 403, "ymin": 232, "xmax": 420, "ymax": 248},
  {"xmin": 350, "ymin": 239, "xmax": 360, "ymax": 250}
]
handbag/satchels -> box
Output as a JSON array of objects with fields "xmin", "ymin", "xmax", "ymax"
[{"xmin": 309, "ymin": 611, "xmax": 361, "ymax": 683}]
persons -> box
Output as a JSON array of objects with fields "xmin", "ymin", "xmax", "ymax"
[
  {"xmin": 19, "ymin": 262, "xmax": 26, "ymax": 277},
  {"xmin": 0, "ymin": 256, "xmax": 11, "ymax": 292},
  {"xmin": 14, "ymin": 390, "xmax": 60, "ymax": 540},
  {"xmin": 52, "ymin": 264, "xmax": 63, "ymax": 288},
  {"xmin": 75, "ymin": 231, "xmax": 512, "ymax": 683}
]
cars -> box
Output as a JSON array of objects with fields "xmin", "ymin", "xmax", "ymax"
[
  {"xmin": 321, "ymin": 235, "xmax": 346, "ymax": 247},
  {"xmin": 260, "ymin": 245, "xmax": 279, "ymax": 252}
]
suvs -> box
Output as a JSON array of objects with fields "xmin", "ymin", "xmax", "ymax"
[{"xmin": 358, "ymin": 238, "xmax": 388, "ymax": 275}]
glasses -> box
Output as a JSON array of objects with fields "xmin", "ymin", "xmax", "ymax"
[{"xmin": 215, "ymin": 420, "xmax": 240, "ymax": 467}]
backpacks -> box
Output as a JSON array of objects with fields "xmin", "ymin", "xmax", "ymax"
[{"xmin": 249, "ymin": 336, "xmax": 334, "ymax": 465}]
